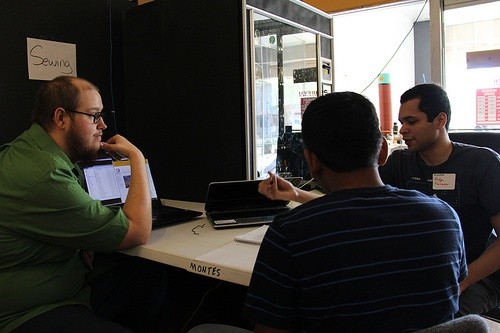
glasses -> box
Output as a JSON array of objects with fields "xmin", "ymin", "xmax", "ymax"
[{"xmin": 65, "ymin": 108, "xmax": 106, "ymax": 124}]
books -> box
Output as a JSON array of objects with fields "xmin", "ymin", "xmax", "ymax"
[{"xmin": 234, "ymin": 225, "xmax": 270, "ymax": 244}]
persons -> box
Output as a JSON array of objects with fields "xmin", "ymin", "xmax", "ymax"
[
  {"xmin": 0, "ymin": 75, "xmax": 152, "ymax": 333},
  {"xmin": 258, "ymin": 84, "xmax": 500, "ymax": 319},
  {"xmin": 241, "ymin": 91, "xmax": 468, "ymax": 333}
]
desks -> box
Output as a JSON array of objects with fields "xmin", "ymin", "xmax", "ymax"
[{"xmin": 117, "ymin": 188, "xmax": 326, "ymax": 287}]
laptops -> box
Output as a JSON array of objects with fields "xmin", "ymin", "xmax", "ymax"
[
  {"xmin": 204, "ymin": 177, "xmax": 303, "ymax": 229},
  {"xmin": 76, "ymin": 157, "xmax": 203, "ymax": 228}
]
